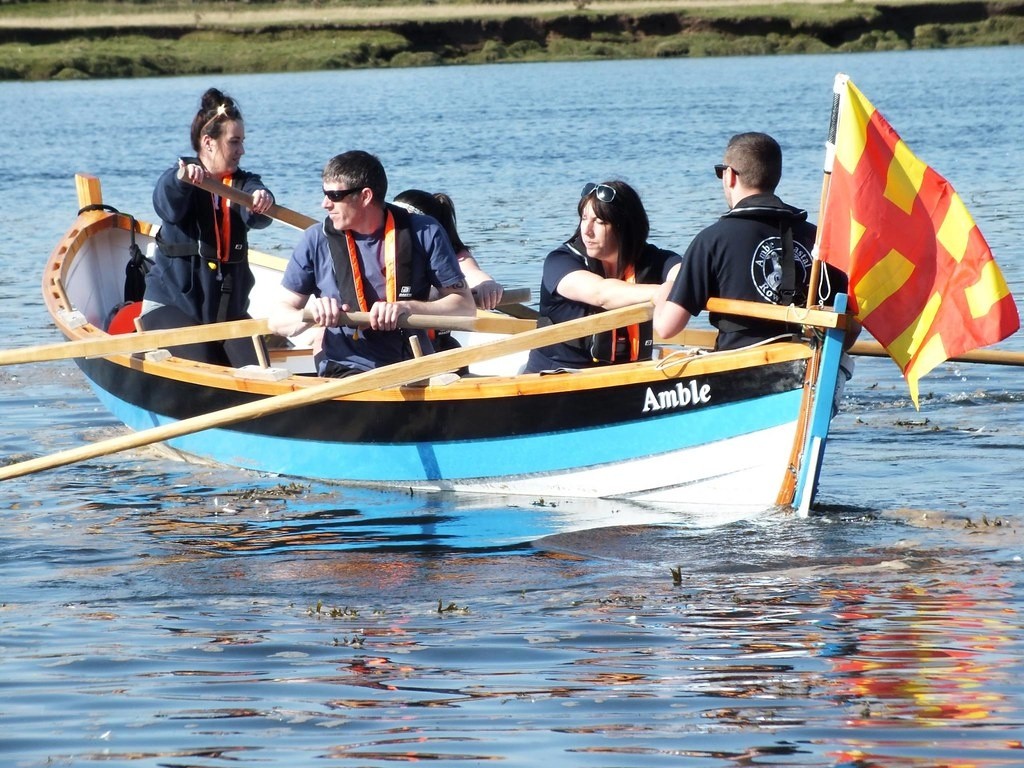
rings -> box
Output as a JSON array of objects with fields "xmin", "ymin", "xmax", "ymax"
[{"xmin": 490, "ymin": 290, "xmax": 496, "ymax": 293}]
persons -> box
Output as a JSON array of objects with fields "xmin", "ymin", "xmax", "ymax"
[
  {"xmin": 269, "ymin": 151, "xmax": 504, "ymax": 386},
  {"xmin": 135, "ymin": 88, "xmax": 275, "ymax": 368},
  {"xmin": 522, "ymin": 181, "xmax": 683, "ymax": 376},
  {"xmin": 652, "ymin": 132, "xmax": 863, "ymax": 406}
]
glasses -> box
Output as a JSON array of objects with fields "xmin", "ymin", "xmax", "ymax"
[
  {"xmin": 321, "ymin": 184, "xmax": 362, "ymax": 203},
  {"xmin": 714, "ymin": 164, "xmax": 740, "ymax": 180},
  {"xmin": 199, "ymin": 103, "xmax": 243, "ymax": 137},
  {"xmin": 580, "ymin": 182, "xmax": 625, "ymax": 210}
]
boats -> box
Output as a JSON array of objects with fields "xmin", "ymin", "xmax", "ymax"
[{"xmin": 39, "ymin": 172, "xmax": 864, "ymax": 517}]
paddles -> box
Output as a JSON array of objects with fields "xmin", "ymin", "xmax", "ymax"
[
  {"xmin": 1, "ymin": 301, "xmax": 655, "ymax": 482},
  {"xmin": 301, "ymin": 302, "xmax": 719, "ymax": 358},
  {"xmin": 845, "ymin": 340, "xmax": 1024, "ymax": 366},
  {"xmin": 176, "ymin": 166, "xmax": 539, "ymax": 320},
  {"xmin": 0, "ymin": 286, "xmax": 533, "ymax": 367}
]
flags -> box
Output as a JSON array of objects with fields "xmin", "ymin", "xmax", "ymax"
[{"xmin": 818, "ymin": 80, "xmax": 1020, "ymax": 412}]
